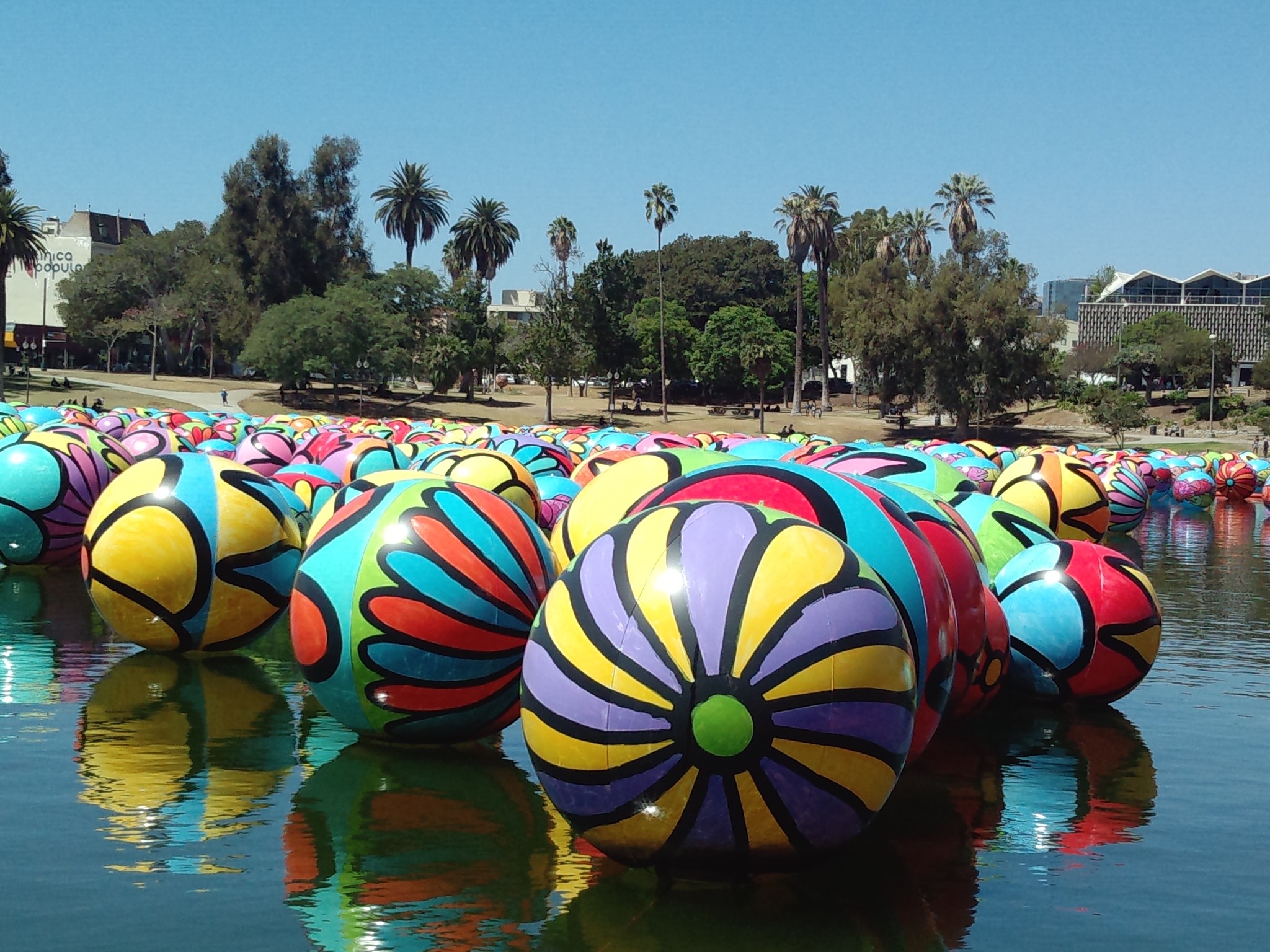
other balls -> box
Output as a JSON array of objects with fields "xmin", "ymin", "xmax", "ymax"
[
  {"xmin": 0, "ymin": 395, "xmax": 1267, "ymax": 883},
  {"xmin": 0, "ymin": 492, "xmax": 1270, "ymax": 951}
]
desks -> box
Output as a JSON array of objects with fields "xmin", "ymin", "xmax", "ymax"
[
  {"xmin": 729, "ymin": 406, "xmax": 752, "ymax": 416},
  {"xmin": 707, "ymin": 405, "xmax": 725, "ymax": 414},
  {"xmin": 885, "ymin": 415, "xmax": 901, "ymax": 422}
]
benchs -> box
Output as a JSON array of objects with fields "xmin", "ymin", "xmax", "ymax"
[
  {"xmin": 881, "ymin": 418, "xmax": 899, "ymax": 423},
  {"xmin": 1229, "ymin": 389, "xmax": 1250, "ymax": 397},
  {"xmin": 1246, "ymin": 430, "xmax": 1265, "ymax": 439},
  {"xmin": 1164, "ymin": 427, "xmax": 1184, "ymax": 438},
  {"xmin": 745, "ymin": 411, "xmax": 751, "ymax": 415},
  {"xmin": 707, "ymin": 409, "xmax": 714, "ymax": 414},
  {"xmin": 720, "ymin": 409, "xmax": 727, "ymax": 414},
  {"xmin": 731, "ymin": 410, "xmax": 738, "ymax": 415}
]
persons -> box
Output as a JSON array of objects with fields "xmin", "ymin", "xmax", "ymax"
[
  {"xmin": 805, "ymin": 400, "xmax": 822, "ymax": 420},
  {"xmin": 1119, "ymin": 371, "xmax": 1228, "ymax": 393},
  {"xmin": 1262, "ymin": 438, "xmax": 1269, "ymax": 459},
  {"xmin": 50, "ymin": 374, "xmax": 70, "ymax": 388},
  {"xmin": 220, "ymin": 389, "xmax": 229, "ymax": 406},
  {"xmin": 766, "ymin": 403, "xmax": 781, "ymax": 412},
  {"xmin": 621, "ymin": 397, "xmax": 651, "ymax": 412},
  {"xmin": 883, "ymin": 402, "xmax": 905, "ymax": 431},
  {"xmin": 57, "ymin": 395, "xmax": 101, "ymax": 413},
  {"xmin": 278, "ymin": 375, "xmax": 312, "ymax": 409},
  {"xmin": 9, "ymin": 362, "xmax": 32, "ymax": 377},
  {"xmin": 781, "ymin": 423, "xmax": 795, "ymax": 436},
  {"xmin": 600, "ymin": 415, "xmax": 609, "ymax": 427},
  {"xmin": 1252, "ymin": 434, "xmax": 1259, "ymax": 454},
  {"xmin": 1168, "ymin": 420, "xmax": 1178, "ymax": 436}
]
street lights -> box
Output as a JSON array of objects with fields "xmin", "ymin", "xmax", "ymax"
[
  {"xmin": 1209, "ymin": 333, "xmax": 1218, "ymax": 438},
  {"xmin": 973, "ymin": 385, "xmax": 987, "ymax": 440}
]
